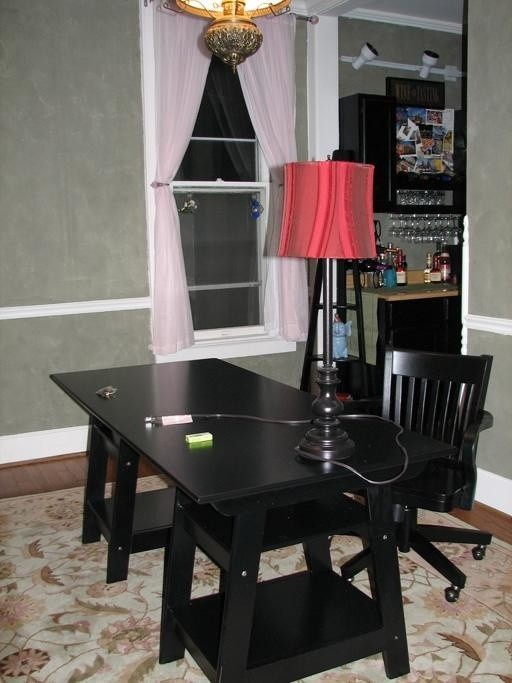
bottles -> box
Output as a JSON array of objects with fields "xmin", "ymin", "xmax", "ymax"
[
  {"xmin": 378, "ymin": 242, "xmax": 408, "ymax": 287},
  {"xmin": 423, "ymin": 241, "xmax": 453, "ymax": 283}
]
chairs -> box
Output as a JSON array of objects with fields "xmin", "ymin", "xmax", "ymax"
[{"xmin": 341, "ymin": 349, "xmax": 492, "ymax": 600}]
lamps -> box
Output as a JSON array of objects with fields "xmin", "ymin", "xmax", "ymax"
[
  {"xmin": 164, "ymin": 0, "xmax": 290, "ymax": 76},
  {"xmin": 271, "ymin": 162, "xmax": 379, "ymax": 463},
  {"xmin": 351, "ymin": 44, "xmax": 439, "ymax": 79}
]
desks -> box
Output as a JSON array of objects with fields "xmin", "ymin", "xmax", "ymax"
[{"xmin": 51, "ymin": 357, "xmax": 459, "ymax": 683}]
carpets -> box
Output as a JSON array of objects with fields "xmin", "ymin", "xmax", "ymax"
[{"xmin": 1, "ymin": 473, "xmax": 509, "ymax": 681}]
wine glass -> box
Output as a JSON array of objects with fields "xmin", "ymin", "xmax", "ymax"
[{"xmin": 386, "ymin": 211, "xmax": 460, "ymax": 246}]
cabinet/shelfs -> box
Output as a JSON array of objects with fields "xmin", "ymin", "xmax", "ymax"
[
  {"xmin": 381, "ymin": 297, "xmax": 463, "ymax": 350},
  {"xmin": 339, "ymin": 97, "xmax": 464, "ymax": 213}
]
